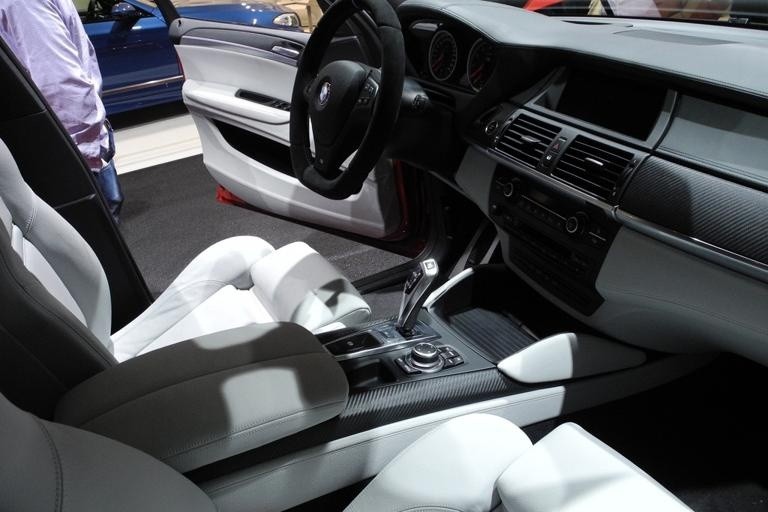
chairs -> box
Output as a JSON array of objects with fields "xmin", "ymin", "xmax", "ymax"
[
  {"xmin": 3, "ymin": 133, "xmax": 377, "ymax": 426},
  {"xmin": 2, "ymin": 391, "xmax": 698, "ymax": 512}
]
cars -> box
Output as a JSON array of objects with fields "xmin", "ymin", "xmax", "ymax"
[{"xmin": 78, "ymin": 0, "xmax": 304, "ymax": 116}]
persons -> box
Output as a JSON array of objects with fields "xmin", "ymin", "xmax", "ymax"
[{"xmin": 0, "ymin": 0, "xmax": 124, "ymax": 229}]
